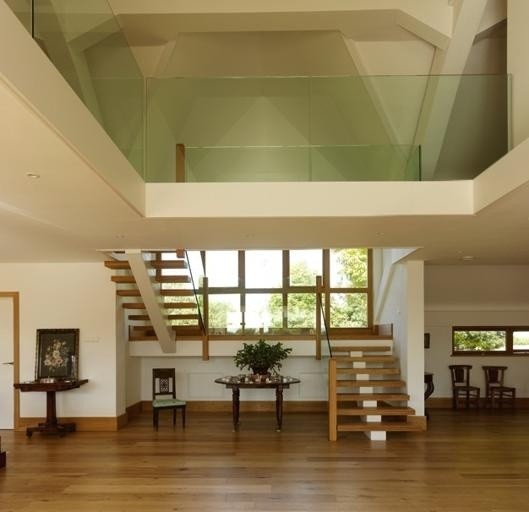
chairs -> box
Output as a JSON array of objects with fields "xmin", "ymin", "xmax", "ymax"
[
  {"xmin": 449, "ymin": 364, "xmax": 515, "ymax": 412},
  {"xmin": 151, "ymin": 367, "xmax": 187, "ymax": 432}
]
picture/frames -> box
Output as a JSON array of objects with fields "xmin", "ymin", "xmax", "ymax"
[{"xmin": 34, "ymin": 327, "xmax": 79, "ymax": 383}]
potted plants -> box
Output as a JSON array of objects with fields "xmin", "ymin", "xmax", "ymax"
[{"xmin": 232, "ymin": 338, "xmax": 293, "ymax": 382}]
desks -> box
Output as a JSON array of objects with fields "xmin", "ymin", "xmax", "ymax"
[
  {"xmin": 214, "ymin": 375, "xmax": 301, "ymax": 434},
  {"xmin": 14, "ymin": 379, "xmax": 88, "ymax": 437}
]
